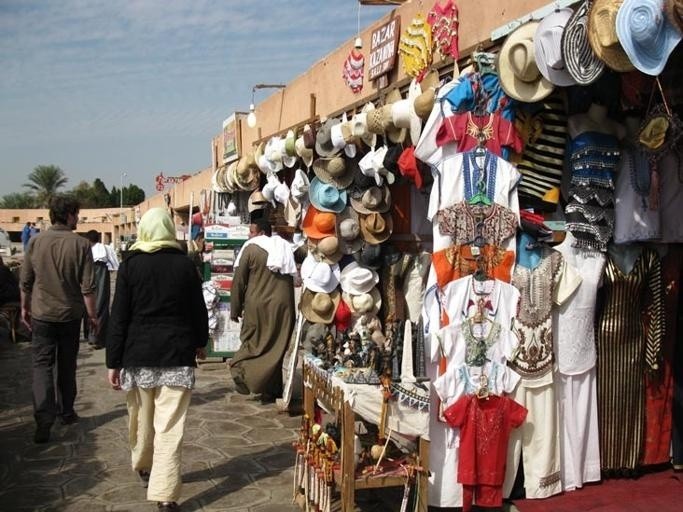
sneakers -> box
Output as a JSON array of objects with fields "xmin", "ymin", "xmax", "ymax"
[
  {"xmin": 157, "ymin": 502, "xmax": 180, "ymax": 511},
  {"xmin": 64, "ymin": 413, "xmax": 78, "ymax": 423},
  {"xmin": 34, "ymin": 420, "xmax": 54, "ymax": 443},
  {"xmin": 139, "ymin": 469, "xmax": 150, "ymax": 489}
]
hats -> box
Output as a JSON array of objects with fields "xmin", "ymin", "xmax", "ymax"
[
  {"xmin": 382, "ymin": 89, "xmax": 407, "ymax": 144},
  {"xmin": 300, "ymin": 102, "xmax": 401, "ymax": 332},
  {"xmin": 397, "ymin": 147, "xmax": 423, "ymax": 189},
  {"xmin": 560, "ymin": 1, "xmax": 606, "ymax": 87},
  {"xmin": 392, "ymin": 77, "xmax": 422, "ymax": 146},
  {"xmin": 616, "ymin": 0, "xmax": 683, "ymax": 77},
  {"xmin": 588, "ymin": 0, "xmax": 637, "ymax": 72},
  {"xmin": 497, "ymin": 19, "xmax": 557, "ymax": 103},
  {"xmin": 212, "ymin": 157, "xmax": 261, "ymax": 194},
  {"xmin": 533, "ymin": 8, "xmax": 578, "ymax": 87},
  {"xmin": 255, "ymin": 123, "xmax": 314, "ymax": 210},
  {"xmin": 414, "ymin": 68, "xmax": 440, "ymax": 125}
]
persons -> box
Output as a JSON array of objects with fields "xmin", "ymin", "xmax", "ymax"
[
  {"xmin": 104, "ymin": 207, "xmax": 210, "ymax": 511},
  {"xmin": 23, "ymin": 196, "xmax": 130, "ymax": 444},
  {"xmin": 229, "ymin": 216, "xmax": 307, "ymax": 405},
  {"xmin": 503, "ymin": 99, "xmax": 683, "ymax": 500}
]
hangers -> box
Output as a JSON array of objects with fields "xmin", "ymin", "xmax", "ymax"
[
  {"xmin": 465, "ymin": 53, "xmax": 499, "ymax": 398},
  {"xmin": 415, "ymin": 1, "xmax": 424, "ymax": 17}
]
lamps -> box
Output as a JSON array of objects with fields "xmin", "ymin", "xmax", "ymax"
[{"xmin": 246, "ymin": 84, "xmax": 287, "ymax": 128}]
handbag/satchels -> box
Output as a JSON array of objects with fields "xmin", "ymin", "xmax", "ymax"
[{"xmin": 633, "ymin": 103, "xmax": 683, "ymax": 165}]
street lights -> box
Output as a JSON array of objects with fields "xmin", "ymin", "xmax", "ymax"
[{"xmin": 121, "ymin": 172, "xmax": 128, "ymax": 214}]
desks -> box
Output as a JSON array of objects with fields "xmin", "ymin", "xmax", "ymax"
[{"xmin": 317, "ymin": 367, "xmax": 430, "ymax": 511}]
